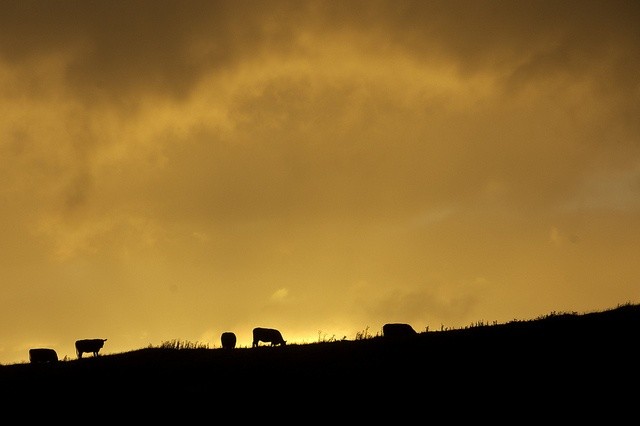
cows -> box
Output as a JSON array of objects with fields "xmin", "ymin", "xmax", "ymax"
[
  {"xmin": 221, "ymin": 332, "xmax": 236, "ymax": 350},
  {"xmin": 75, "ymin": 339, "xmax": 107, "ymax": 359},
  {"xmin": 252, "ymin": 327, "xmax": 287, "ymax": 348},
  {"xmin": 383, "ymin": 323, "xmax": 419, "ymax": 336},
  {"xmin": 29, "ymin": 348, "xmax": 58, "ymax": 364}
]
